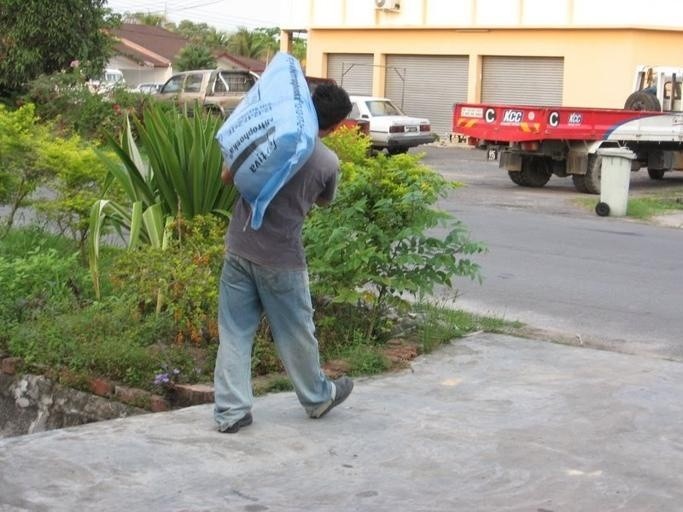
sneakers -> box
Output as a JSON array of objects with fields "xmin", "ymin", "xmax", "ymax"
[
  {"xmin": 219, "ymin": 413, "xmax": 251, "ymax": 433},
  {"xmin": 311, "ymin": 377, "xmax": 353, "ymax": 419}
]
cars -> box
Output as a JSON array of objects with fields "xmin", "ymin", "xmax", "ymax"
[{"xmin": 347, "ymin": 96, "xmax": 436, "ymax": 155}]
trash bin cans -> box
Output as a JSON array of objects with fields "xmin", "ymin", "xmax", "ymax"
[{"xmin": 595, "ymin": 146, "xmax": 637, "ymax": 216}]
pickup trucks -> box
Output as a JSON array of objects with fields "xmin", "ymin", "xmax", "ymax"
[{"xmin": 140, "ymin": 68, "xmax": 262, "ymax": 121}]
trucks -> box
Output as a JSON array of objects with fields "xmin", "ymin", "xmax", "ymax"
[{"xmin": 452, "ymin": 64, "xmax": 683, "ymax": 194}]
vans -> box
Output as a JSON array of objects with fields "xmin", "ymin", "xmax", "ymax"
[{"xmin": 81, "ymin": 69, "xmax": 124, "ymax": 92}]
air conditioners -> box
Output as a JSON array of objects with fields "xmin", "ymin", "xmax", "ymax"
[{"xmin": 372, "ymin": 0, "xmax": 402, "ymax": 14}]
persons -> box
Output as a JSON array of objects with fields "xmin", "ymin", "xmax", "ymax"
[{"xmin": 213, "ymin": 84, "xmax": 354, "ymax": 432}]
